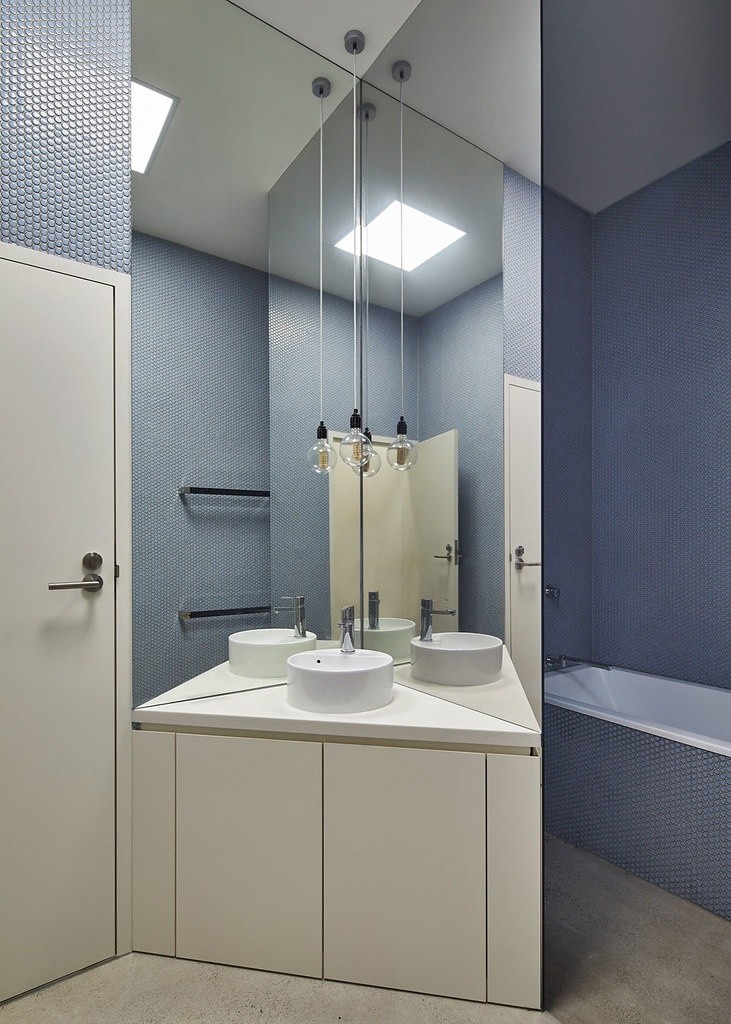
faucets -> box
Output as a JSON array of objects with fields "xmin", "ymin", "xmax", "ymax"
[
  {"xmin": 545, "ymin": 654, "xmax": 613, "ymax": 673},
  {"xmin": 337, "ymin": 604, "xmax": 355, "ymax": 654},
  {"xmin": 368, "ymin": 589, "xmax": 381, "ymax": 630},
  {"xmin": 419, "ymin": 598, "xmax": 457, "ymax": 642},
  {"xmin": 273, "ymin": 596, "xmax": 307, "ymax": 639}
]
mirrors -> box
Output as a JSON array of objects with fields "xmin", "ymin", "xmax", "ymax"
[{"xmin": 131, "ymin": 0, "xmax": 543, "ymax": 730}]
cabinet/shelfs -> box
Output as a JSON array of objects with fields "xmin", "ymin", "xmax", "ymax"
[{"xmin": 131, "ymin": 728, "xmax": 543, "ymax": 1009}]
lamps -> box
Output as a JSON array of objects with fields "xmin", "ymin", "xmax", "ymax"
[
  {"xmin": 339, "ymin": 29, "xmax": 373, "ymax": 467},
  {"xmin": 350, "ymin": 101, "xmax": 381, "ymax": 477},
  {"xmin": 305, "ymin": 75, "xmax": 339, "ymax": 480},
  {"xmin": 386, "ymin": 61, "xmax": 417, "ymax": 469}
]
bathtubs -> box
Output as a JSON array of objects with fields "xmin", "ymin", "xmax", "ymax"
[{"xmin": 542, "ymin": 663, "xmax": 731, "ymax": 925}]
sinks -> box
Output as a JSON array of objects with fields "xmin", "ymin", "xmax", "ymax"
[
  {"xmin": 353, "ymin": 617, "xmax": 416, "ymax": 658},
  {"xmin": 228, "ymin": 629, "xmax": 317, "ymax": 678},
  {"xmin": 286, "ymin": 648, "xmax": 393, "ymax": 714},
  {"xmin": 410, "ymin": 632, "xmax": 504, "ymax": 686}
]
